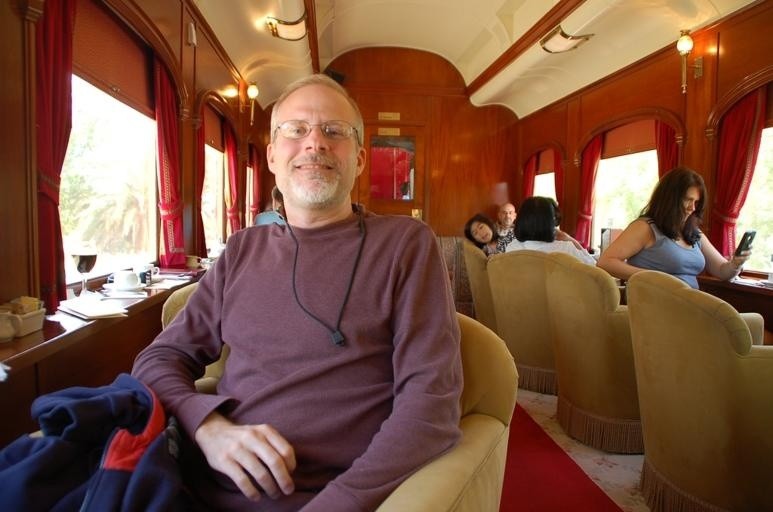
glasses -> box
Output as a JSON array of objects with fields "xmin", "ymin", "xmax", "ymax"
[{"xmin": 271, "ymin": 119, "xmax": 362, "ymax": 146}]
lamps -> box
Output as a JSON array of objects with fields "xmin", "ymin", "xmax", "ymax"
[
  {"xmin": 677, "ymin": 29, "xmax": 702, "ymax": 94},
  {"xmin": 240, "ymin": 82, "xmax": 259, "ymax": 125}
]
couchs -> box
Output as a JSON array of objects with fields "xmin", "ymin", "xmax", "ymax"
[
  {"xmin": 483, "ymin": 248, "xmax": 553, "ymax": 395},
  {"xmin": 464, "ymin": 239, "xmax": 493, "ymax": 333},
  {"xmin": 160, "ymin": 276, "xmax": 202, "ymax": 330},
  {"xmin": 625, "ymin": 269, "xmax": 772, "ymax": 510},
  {"xmin": 544, "ymin": 251, "xmax": 646, "ymax": 460},
  {"xmin": 166, "ymin": 308, "xmax": 521, "ymax": 510}
]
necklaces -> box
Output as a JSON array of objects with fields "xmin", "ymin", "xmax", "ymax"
[{"xmin": 281, "ymin": 213, "xmax": 368, "ymax": 345}]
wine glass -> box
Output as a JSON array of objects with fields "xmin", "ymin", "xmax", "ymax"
[{"xmin": 70, "ymin": 240, "xmax": 98, "ymax": 298}]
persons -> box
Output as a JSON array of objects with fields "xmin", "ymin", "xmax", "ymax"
[
  {"xmin": 252, "ymin": 186, "xmax": 287, "ymax": 226},
  {"xmin": 128, "ymin": 74, "xmax": 463, "ymax": 511},
  {"xmin": 596, "ymin": 165, "xmax": 753, "ymax": 290},
  {"xmin": 463, "ymin": 213, "xmax": 510, "ymax": 255},
  {"xmin": 507, "ymin": 196, "xmax": 597, "ymax": 266},
  {"xmin": 492, "ymin": 202, "xmax": 517, "ymax": 237}
]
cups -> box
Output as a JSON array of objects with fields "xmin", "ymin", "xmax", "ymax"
[
  {"xmin": 0, "ymin": 310, "xmax": 23, "ymax": 343},
  {"xmin": 183, "ymin": 255, "xmax": 202, "ymax": 271},
  {"xmin": 108, "ymin": 271, "xmax": 141, "ymax": 286},
  {"xmin": 132, "ymin": 263, "xmax": 160, "ymax": 279}
]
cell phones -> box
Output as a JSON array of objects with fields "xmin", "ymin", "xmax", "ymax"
[{"xmin": 735, "ymin": 231, "xmax": 756, "ymax": 256}]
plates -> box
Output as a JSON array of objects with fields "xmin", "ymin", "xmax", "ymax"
[{"xmin": 102, "ymin": 283, "xmax": 147, "ymax": 293}]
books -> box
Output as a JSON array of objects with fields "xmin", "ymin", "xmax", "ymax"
[{"xmin": 57, "ymin": 297, "xmax": 129, "ymax": 320}]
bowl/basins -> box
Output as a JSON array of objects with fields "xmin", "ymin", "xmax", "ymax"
[{"xmin": 10, "ymin": 308, "xmax": 46, "ymax": 338}]
